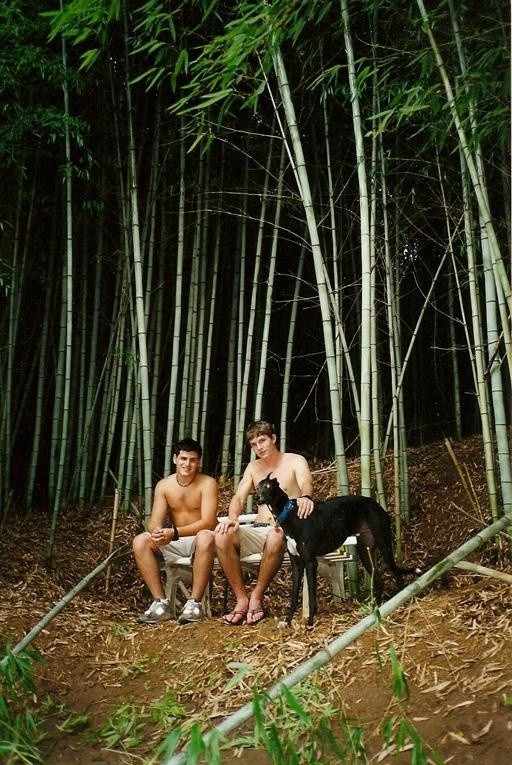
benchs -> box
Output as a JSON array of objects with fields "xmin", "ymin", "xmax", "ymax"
[{"xmin": 165, "ymin": 515, "xmax": 357, "ymax": 620}]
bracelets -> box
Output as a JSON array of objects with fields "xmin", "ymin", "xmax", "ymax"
[
  {"xmin": 300, "ymin": 495, "xmax": 314, "ymax": 501},
  {"xmin": 172, "ymin": 525, "xmax": 179, "ymax": 542}
]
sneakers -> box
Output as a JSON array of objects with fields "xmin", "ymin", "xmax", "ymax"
[
  {"xmin": 137, "ymin": 598, "xmax": 174, "ymax": 623},
  {"xmin": 177, "ymin": 598, "xmax": 203, "ymax": 624}
]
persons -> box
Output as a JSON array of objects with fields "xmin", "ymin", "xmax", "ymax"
[
  {"xmin": 132, "ymin": 437, "xmax": 217, "ymax": 624},
  {"xmin": 214, "ymin": 420, "xmax": 315, "ymax": 625}
]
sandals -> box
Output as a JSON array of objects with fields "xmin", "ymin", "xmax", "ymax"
[{"xmin": 224, "ymin": 608, "xmax": 264, "ymax": 625}]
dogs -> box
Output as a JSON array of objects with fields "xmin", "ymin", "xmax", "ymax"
[{"xmin": 252, "ymin": 471, "xmax": 416, "ymax": 629}]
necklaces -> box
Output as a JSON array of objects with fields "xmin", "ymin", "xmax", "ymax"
[{"xmin": 175, "ymin": 473, "xmax": 199, "ymax": 486}]
